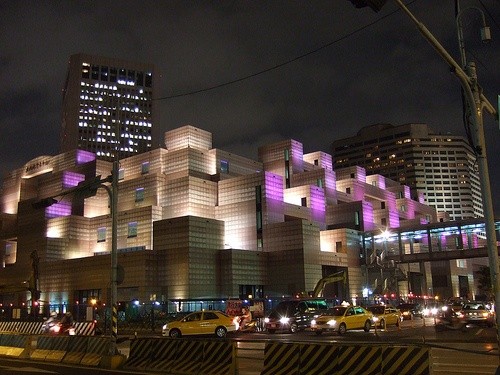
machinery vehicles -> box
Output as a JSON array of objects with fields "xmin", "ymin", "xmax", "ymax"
[{"xmin": 306, "ymin": 269, "xmax": 348, "ymax": 302}]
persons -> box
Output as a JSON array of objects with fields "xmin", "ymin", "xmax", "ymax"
[
  {"xmin": 45, "ymin": 311, "xmax": 73, "ymax": 333},
  {"xmin": 238, "ymin": 307, "xmax": 252, "ymax": 330}
]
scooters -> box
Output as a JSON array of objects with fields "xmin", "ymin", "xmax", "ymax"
[
  {"xmin": 231, "ymin": 316, "xmax": 257, "ymax": 334},
  {"xmin": 58, "ymin": 321, "xmax": 77, "ymax": 336},
  {"xmin": 38, "ymin": 316, "xmax": 63, "ymax": 335}
]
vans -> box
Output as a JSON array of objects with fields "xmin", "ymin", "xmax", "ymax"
[{"xmin": 264, "ymin": 296, "xmax": 329, "ymax": 334}]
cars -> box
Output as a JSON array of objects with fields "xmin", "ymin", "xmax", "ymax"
[
  {"xmin": 161, "ymin": 308, "xmax": 240, "ymax": 338},
  {"xmin": 364, "ymin": 303, "xmax": 404, "ymax": 329},
  {"xmin": 394, "ymin": 294, "xmax": 497, "ymax": 331},
  {"xmin": 310, "ymin": 300, "xmax": 376, "ymax": 336}
]
